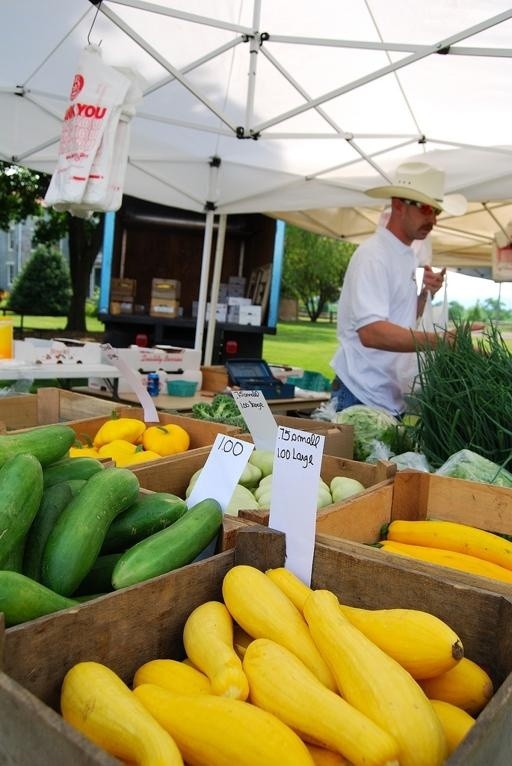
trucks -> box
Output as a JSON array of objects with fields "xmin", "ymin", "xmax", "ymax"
[{"xmin": 97, "ymin": 203, "xmax": 288, "ymax": 359}]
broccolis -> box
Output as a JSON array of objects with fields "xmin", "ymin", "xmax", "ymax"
[{"xmin": 191, "ymin": 394, "xmax": 246, "ymax": 431}]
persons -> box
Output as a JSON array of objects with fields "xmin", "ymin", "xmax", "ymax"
[{"xmin": 327, "ymin": 163, "xmax": 486, "ymax": 415}]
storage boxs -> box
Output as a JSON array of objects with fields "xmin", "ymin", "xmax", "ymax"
[
  {"xmin": 149, "ymin": 299, "xmax": 180, "ymax": 317},
  {"xmin": 192, "ymin": 275, "xmax": 262, "ymax": 325},
  {"xmin": 109, "ymin": 276, "xmax": 136, "ymax": 302},
  {"xmin": 151, "ymin": 277, "xmax": 181, "ymax": 299}
]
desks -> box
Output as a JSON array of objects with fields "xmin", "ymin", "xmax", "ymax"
[
  {"xmin": 70, "ymin": 385, "xmax": 330, "ymax": 417},
  {"xmin": 1, "ymin": 340, "xmax": 122, "ymax": 400}
]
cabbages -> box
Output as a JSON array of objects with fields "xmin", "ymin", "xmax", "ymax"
[{"xmin": 335, "ymin": 405, "xmax": 399, "ymax": 460}]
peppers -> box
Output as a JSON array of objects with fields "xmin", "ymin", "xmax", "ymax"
[{"xmin": 69, "ymin": 418, "xmax": 190, "ymax": 468}]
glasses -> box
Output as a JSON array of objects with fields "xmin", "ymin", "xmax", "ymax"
[{"xmin": 399, "ymin": 197, "xmax": 442, "ymax": 214}]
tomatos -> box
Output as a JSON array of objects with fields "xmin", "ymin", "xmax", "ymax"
[{"xmin": 185, "ymin": 449, "xmax": 364, "ymax": 518}]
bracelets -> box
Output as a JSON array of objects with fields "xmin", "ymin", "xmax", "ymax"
[{"xmin": 439, "ymin": 332, "xmax": 445, "ymax": 341}]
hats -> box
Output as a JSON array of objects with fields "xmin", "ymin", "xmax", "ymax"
[{"xmin": 364, "ymin": 161, "xmax": 469, "ymax": 216}]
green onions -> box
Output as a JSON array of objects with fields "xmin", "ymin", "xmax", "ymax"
[{"xmin": 395, "ymin": 316, "xmax": 512, "ymax": 487}]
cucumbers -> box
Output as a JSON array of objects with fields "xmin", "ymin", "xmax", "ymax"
[
  {"xmin": 0, "ymin": 425, "xmax": 221, "ymax": 626},
  {"xmin": 369, "ymin": 519, "xmax": 512, "ymax": 585},
  {"xmin": 61, "ymin": 565, "xmax": 494, "ymax": 766}
]
